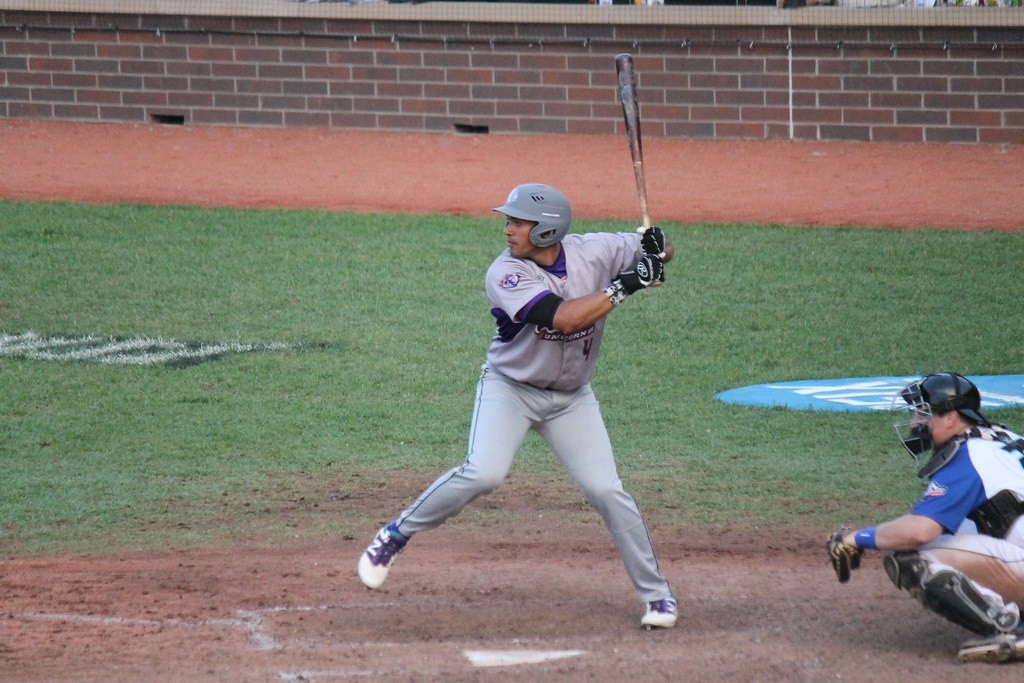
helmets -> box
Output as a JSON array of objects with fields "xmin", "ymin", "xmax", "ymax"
[
  {"xmin": 491, "ymin": 184, "xmax": 571, "ymax": 248},
  {"xmin": 900, "ymin": 372, "xmax": 991, "ymax": 429}
]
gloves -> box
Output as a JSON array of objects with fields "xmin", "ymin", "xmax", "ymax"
[
  {"xmin": 637, "ymin": 225, "xmax": 666, "ymax": 253},
  {"xmin": 618, "ymin": 253, "xmax": 668, "ymax": 296}
]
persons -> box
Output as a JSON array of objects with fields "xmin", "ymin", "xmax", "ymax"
[
  {"xmin": 357, "ymin": 184, "xmax": 678, "ymax": 630},
  {"xmin": 829, "ymin": 372, "xmax": 1024, "ymax": 664}
]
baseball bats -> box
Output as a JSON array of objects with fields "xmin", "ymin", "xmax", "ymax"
[{"xmin": 615, "ymin": 53, "xmax": 663, "ymax": 287}]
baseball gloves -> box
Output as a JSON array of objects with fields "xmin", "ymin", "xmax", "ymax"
[{"xmin": 827, "ymin": 528, "xmax": 861, "ymax": 584}]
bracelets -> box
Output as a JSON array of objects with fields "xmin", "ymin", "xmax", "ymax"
[{"xmin": 855, "ymin": 527, "xmax": 878, "ymax": 550}]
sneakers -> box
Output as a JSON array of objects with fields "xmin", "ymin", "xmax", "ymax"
[
  {"xmin": 957, "ymin": 631, "xmax": 1024, "ymax": 663},
  {"xmin": 357, "ymin": 522, "xmax": 407, "ymax": 591},
  {"xmin": 641, "ymin": 594, "xmax": 678, "ymax": 631}
]
